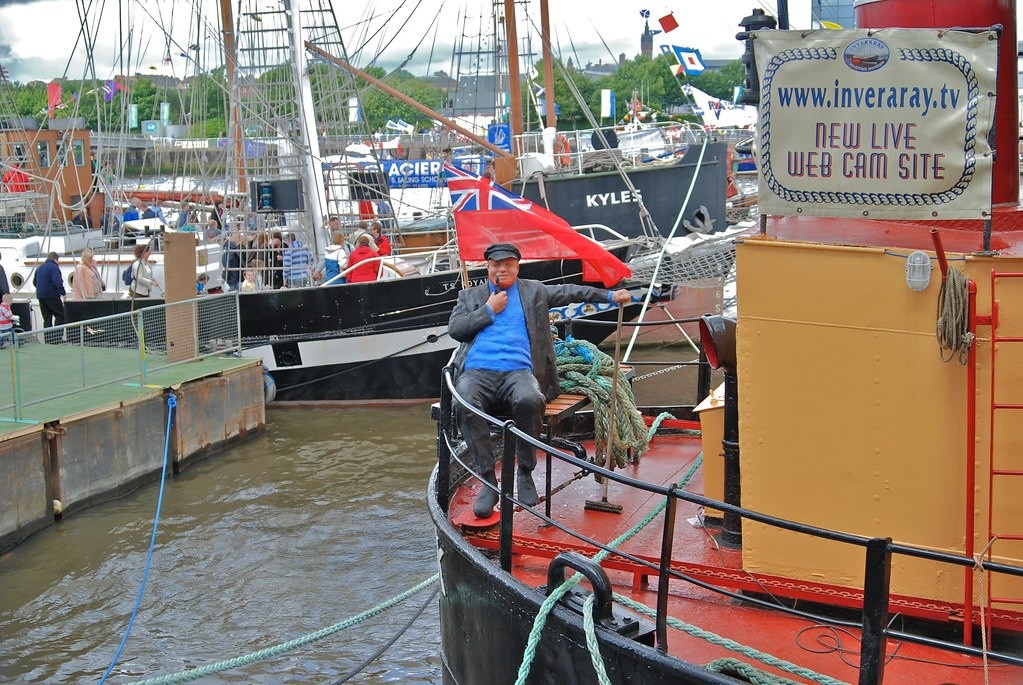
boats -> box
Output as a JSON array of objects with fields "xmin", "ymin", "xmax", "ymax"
[{"xmin": 427, "ymin": 0, "xmax": 1023, "ymax": 685}]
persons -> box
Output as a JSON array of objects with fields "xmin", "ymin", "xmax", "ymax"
[
  {"xmin": 5, "ymin": 145, "xmax": 225, "ymax": 244},
  {"xmin": 33, "ymin": 252, "xmax": 66, "ymax": 345},
  {"xmin": 129, "ymin": 246, "xmax": 158, "ymax": 297},
  {"xmin": 221, "ymin": 217, "xmax": 391, "ymax": 292},
  {"xmin": 355, "ymin": 125, "xmax": 461, "ymax": 144},
  {"xmin": 448, "ymin": 243, "xmax": 629, "ymax": 518},
  {"xmin": 0, "ymin": 253, "xmax": 20, "ymax": 350},
  {"xmin": 196, "ymin": 274, "xmax": 209, "ymax": 296},
  {"xmin": 73, "ymin": 247, "xmax": 105, "ymax": 300},
  {"xmin": 373, "ymin": 201, "xmax": 399, "ymax": 255}
]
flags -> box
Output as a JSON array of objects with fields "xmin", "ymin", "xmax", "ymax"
[
  {"xmin": 445, "ymin": 162, "xmax": 633, "ymax": 288},
  {"xmin": 86, "ymin": 80, "xmax": 129, "ymax": 103},
  {"xmin": 672, "ymin": 44, "xmax": 707, "ymax": 78}
]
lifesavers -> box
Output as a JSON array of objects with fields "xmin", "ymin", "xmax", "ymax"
[
  {"xmin": 555, "ymin": 133, "xmax": 571, "ymax": 164},
  {"xmin": 264, "ymin": 374, "xmax": 277, "ymax": 404}
]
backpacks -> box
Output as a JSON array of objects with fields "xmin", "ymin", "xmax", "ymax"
[{"xmin": 122, "ymin": 260, "xmax": 143, "ymax": 285}]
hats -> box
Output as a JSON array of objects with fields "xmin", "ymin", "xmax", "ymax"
[{"xmin": 484, "ymin": 243, "xmax": 521, "ymax": 261}]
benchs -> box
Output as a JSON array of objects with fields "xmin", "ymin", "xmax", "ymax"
[{"xmin": 432, "ymin": 365, "xmax": 636, "ymax": 526}]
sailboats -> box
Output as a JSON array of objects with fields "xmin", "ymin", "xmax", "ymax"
[{"xmin": 0, "ymin": 0, "xmax": 758, "ymax": 404}]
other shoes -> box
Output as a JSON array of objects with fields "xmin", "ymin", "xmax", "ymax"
[
  {"xmin": 473, "ymin": 473, "xmax": 499, "ymax": 518},
  {"xmin": 517, "ymin": 469, "xmax": 538, "ymax": 506}
]
